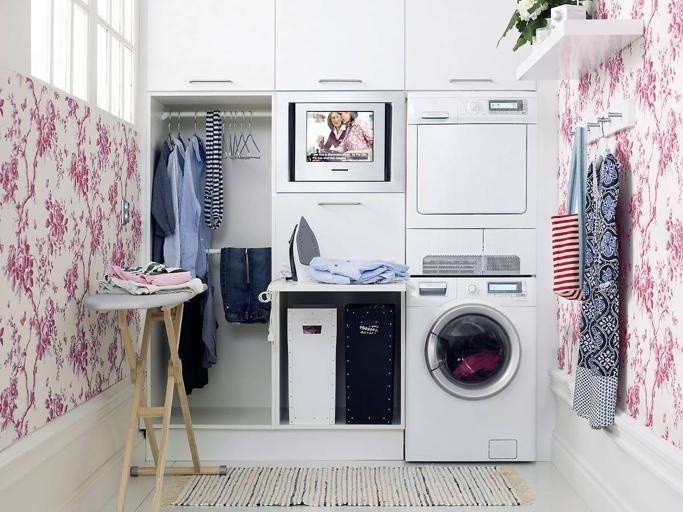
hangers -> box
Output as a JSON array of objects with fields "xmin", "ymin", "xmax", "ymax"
[{"xmin": 163, "ymin": 107, "xmax": 263, "ymax": 163}]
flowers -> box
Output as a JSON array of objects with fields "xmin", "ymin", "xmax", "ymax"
[{"xmin": 496, "ymin": 0, "xmax": 597, "ymax": 51}]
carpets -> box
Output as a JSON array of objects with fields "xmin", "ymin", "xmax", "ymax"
[{"xmin": 169, "ymin": 463, "xmax": 522, "ymax": 508}]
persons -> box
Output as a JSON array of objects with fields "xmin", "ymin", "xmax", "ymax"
[
  {"xmin": 315, "ymin": 111, "xmax": 346, "ymax": 151},
  {"xmin": 332, "ymin": 111, "xmax": 373, "ymax": 153}
]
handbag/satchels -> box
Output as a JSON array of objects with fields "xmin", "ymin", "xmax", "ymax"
[{"xmin": 551, "ymin": 213, "xmax": 587, "ymax": 301}]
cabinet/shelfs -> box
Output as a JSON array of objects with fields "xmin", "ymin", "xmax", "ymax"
[
  {"xmin": 274, "ymin": 0, "xmax": 406, "ymax": 93},
  {"xmin": 406, "ymin": 2, "xmax": 536, "ymax": 90},
  {"xmin": 271, "ymin": 193, "xmax": 407, "ymax": 270},
  {"xmin": 137, "ymin": 0, "xmax": 274, "ymax": 93}
]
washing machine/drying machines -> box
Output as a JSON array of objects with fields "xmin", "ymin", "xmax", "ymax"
[
  {"xmin": 406, "ymin": 90, "xmax": 540, "ymax": 277},
  {"xmin": 404, "ymin": 274, "xmax": 537, "ymax": 466}
]
